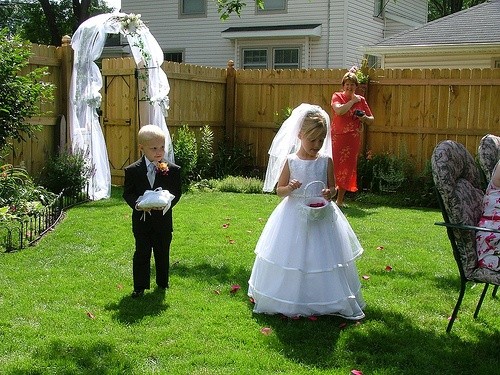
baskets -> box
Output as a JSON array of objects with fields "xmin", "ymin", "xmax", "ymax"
[{"xmin": 298, "ymin": 181, "xmax": 330, "ymax": 224}]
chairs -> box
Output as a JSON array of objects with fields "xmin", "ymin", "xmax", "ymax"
[{"xmin": 429, "ymin": 132, "xmax": 500, "ymax": 334}]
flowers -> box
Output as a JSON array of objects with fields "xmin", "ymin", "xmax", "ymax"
[
  {"xmin": 350, "ymin": 53, "xmax": 379, "ymax": 84},
  {"xmin": 155, "ymin": 162, "xmax": 171, "ymax": 179},
  {"xmin": 120, "ymin": 13, "xmax": 153, "ymax": 106}
]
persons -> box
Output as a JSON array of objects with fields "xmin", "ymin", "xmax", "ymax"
[
  {"xmin": 329, "ymin": 72, "xmax": 375, "ymax": 208},
  {"xmin": 476, "ymin": 158, "xmax": 500, "ymax": 274},
  {"xmin": 123, "ymin": 123, "xmax": 181, "ymax": 298},
  {"xmin": 247, "ymin": 109, "xmax": 367, "ymax": 320}
]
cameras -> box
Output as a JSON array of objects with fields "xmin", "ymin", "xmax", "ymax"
[{"xmin": 354, "ymin": 110, "xmax": 364, "ymax": 117}]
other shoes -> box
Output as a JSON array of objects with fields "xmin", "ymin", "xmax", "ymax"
[
  {"xmin": 334, "ymin": 201, "xmax": 352, "ymax": 208},
  {"xmin": 131, "ymin": 282, "xmax": 171, "ymax": 299}
]
